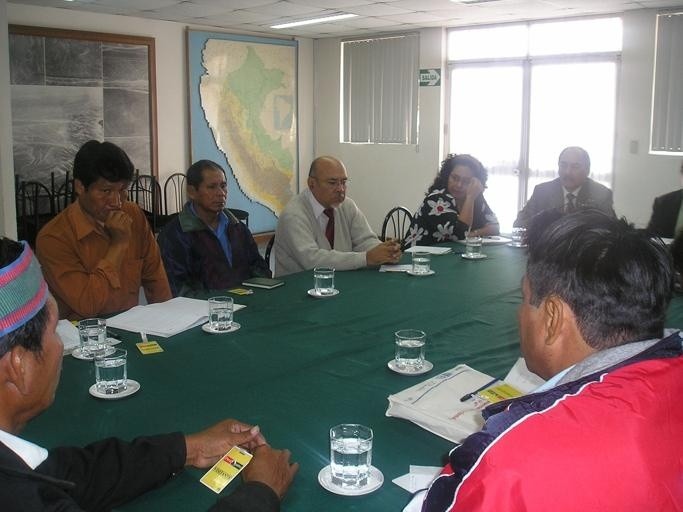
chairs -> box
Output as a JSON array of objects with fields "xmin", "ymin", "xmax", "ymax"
[
  {"xmin": 381, "ymin": 206, "xmax": 414, "ymax": 248},
  {"xmin": 13, "ymin": 167, "xmax": 277, "ymax": 289}
]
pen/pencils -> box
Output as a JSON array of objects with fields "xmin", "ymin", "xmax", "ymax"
[
  {"xmin": 106, "ymin": 330, "xmax": 121, "ymax": 339},
  {"xmin": 461, "ymin": 377, "xmax": 503, "ymax": 404}
]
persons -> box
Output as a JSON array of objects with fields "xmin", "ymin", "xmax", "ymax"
[
  {"xmin": 275, "ymin": 157, "xmax": 402, "ymax": 272},
  {"xmin": 156, "ymin": 160, "xmax": 272, "ymax": 294},
  {"xmin": 510, "ymin": 147, "xmax": 615, "ymax": 234},
  {"xmin": 422, "ymin": 207, "xmax": 682, "ymax": 512},
  {"xmin": 402, "ymin": 154, "xmax": 500, "ymax": 245},
  {"xmin": 35, "ymin": 139, "xmax": 171, "ymax": 318},
  {"xmin": 644, "ymin": 160, "xmax": 683, "ymax": 238},
  {"xmin": 1, "ymin": 233, "xmax": 298, "ymax": 512}
]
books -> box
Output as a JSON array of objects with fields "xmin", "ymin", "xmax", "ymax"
[
  {"xmin": 105, "ymin": 296, "xmax": 247, "ymax": 338},
  {"xmin": 385, "ymin": 361, "xmax": 526, "ymax": 449},
  {"xmin": 240, "ymin": 276, "xmax": 285, "ymax": 290}
]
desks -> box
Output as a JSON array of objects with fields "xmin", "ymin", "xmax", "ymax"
[{"xmin": 18, "ymin": 232, "xmax": 682, "ymax": 511}]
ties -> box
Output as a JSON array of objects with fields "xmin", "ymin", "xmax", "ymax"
[
  {"xmin": 565, "ymin": 194, "xmax": 577, "ymax": 208},
  {"xmin": 324, "ymin": 208, "xmax": 335, "ymax": 250}
]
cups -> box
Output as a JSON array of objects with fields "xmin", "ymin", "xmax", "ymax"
[
  {"xmin": 329, "ymin": 423, "xmax": 374, "ymax": 488},
  {"xmin": 466, "ymin": 237, "xmax": 482, "ymax": 258},
  {"xmin": 208, "ymin": 296, "xmax": 233, "ymax": 330},
  {"xmin": 511, "ymin": 228, "xmax": 526, "ymax": 244},
  {"xmin": 313, "ymin": 267, "xmax": 335, "ymax": 294},
  {"xmin": 78, "ymin": 318, "xmax": 107, "ymax": 355},
  {"xmin": 95, "ymin": 349, "xmax": 128, "ymax": 393},
  {"xmin": 396, "ymin": 329, "xmax": 427, "ymax": 372},
  {"xmin": 411, "ymin": 251, "xmax": 430, "ymax": 273}
]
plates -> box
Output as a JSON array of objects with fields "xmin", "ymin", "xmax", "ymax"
[
  {"xmin": 388, "ymin": 358, "xmax": 434, "ymax": 376},
  {"xmin": 318, "ymin": 465, "xmax": 384, "ymax": 496},
  {"xmin": 202, "ymin": 321, "xmax": 240, "ymax": 334},
  {"xmin": 72, "ymin": 345, "xmax": 117, "ymax": 361},
  {"xmin": 308, "ymin": 287, "xmax": 339, "ymax": 299},
  {"xmin": 408, "ymin": 269, "xmax": 436, "ymax": 277},
  {"xmin": 462, "ymin": 253, "xmax": 488, "ymax": 260},
  {"xmin": 89, "ymin": 379, "xmax": 142, "ymax": 401},
  {"xmin": 507, "ymin": 243, "xmax": 527, "ymax": 248}
]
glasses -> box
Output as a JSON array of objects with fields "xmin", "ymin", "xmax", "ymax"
[{"xmin": 318, "ymin": 178, "xmax": 352, "ymax": 186}]
